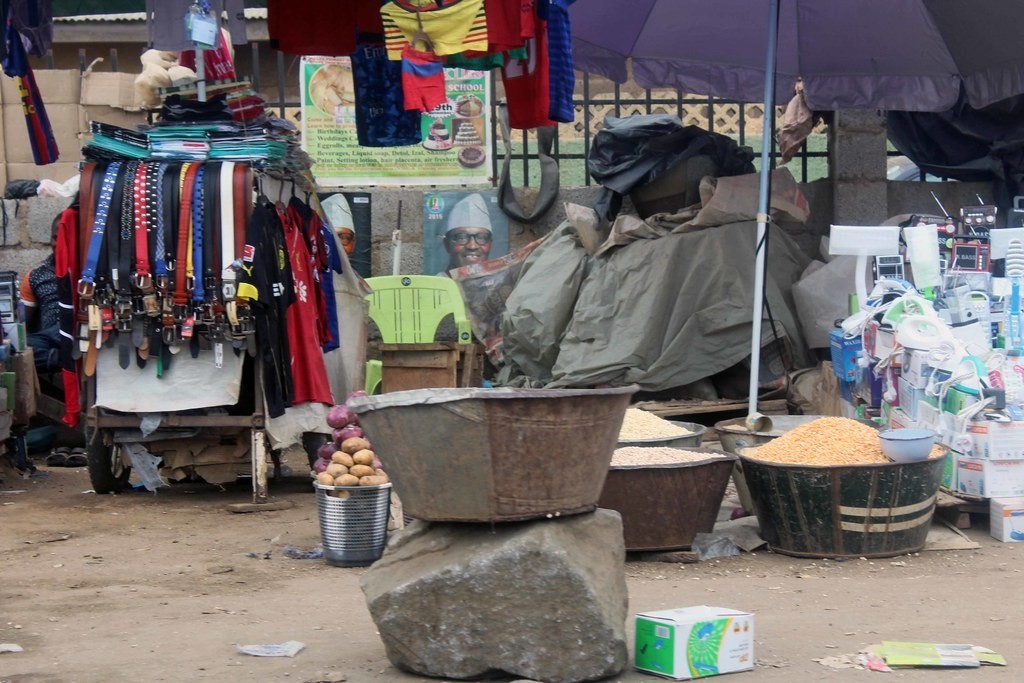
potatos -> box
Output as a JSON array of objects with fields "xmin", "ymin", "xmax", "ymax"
[{"xmin": 316, "ymin": 436, "xmax": 388, "ymax": 498}]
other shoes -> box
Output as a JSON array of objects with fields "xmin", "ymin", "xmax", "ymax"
[
  {"xmin": 47, "ymin": 446, "xmax": 71, "ymax": 466},
  {"xmin": 66, "ymin": 447, "xmax": 89, "ymax": 467}
]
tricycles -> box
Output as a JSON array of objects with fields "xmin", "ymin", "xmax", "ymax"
[{"xmin": 78, "ymin": 160, "xmax": 335, "ymax": 495}]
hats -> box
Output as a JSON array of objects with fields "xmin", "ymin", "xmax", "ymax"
[
  {"xmin": 319, "ymin": 193, "xmax": 354, "ymax": 232},
  {"xmin": 444, "ymin": 193, "xmax": 492, "ymax": 235}
]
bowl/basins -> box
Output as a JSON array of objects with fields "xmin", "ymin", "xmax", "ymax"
[
  {"xmin": 597, "ymin": 446, "xmax": 739, "ymax": 553},
  {"xmin": 616, "ymin": 420, "xmax": 708, "ymax": 447},
  {"xmin": 734, "ymin": 443, "xmax": 950, "ymax": 558},
  {"xmin": 877, "ymin": 426, "xmax": 935, "ymax": 463},
  {"xmin": 346, "ymin": 382, "xmax": 641, "ymax": 524},
  {"xmin": 715, "ymin": 413, "xmax": 828, "ymax": 515}
]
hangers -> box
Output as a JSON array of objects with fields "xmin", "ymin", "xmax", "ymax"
[{"xmin": 405, "ymin": 11, "xmax": 437, "ymax": 52}]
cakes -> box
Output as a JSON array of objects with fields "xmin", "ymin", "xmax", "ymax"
[
  {"xmin": 458, "ymin": 147, "xmax": 485, "ymax": 168},
  {"xmin": 454, "ymin": 122, "xmax": 482, "ymax": 144},
  {"xmin": 455, "ymin": 94, "xmax": 483, "ymax": 117},
  {"xmin": 423, "ymin": 118, "xmax": 454, "ymax": 149}
]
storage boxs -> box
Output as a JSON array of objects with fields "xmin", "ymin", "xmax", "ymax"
[
  {"xmin": 633, "ymin": 604, "xmax": 757, "ymax": 682},
  {"xmin": 827, "ymin": 312, "xmax": 1024, "ymax": 546},
  {"xmin": 0, "ymin": 271, "xmax": 43, "ymax": 443}
]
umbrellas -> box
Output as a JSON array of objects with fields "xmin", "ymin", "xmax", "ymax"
[{"xmin": 565, "ymin": 0, "xmax": 1024, "ymax": 415}]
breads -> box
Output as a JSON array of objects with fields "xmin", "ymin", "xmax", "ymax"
[{"xmin": 311, "ymin": 65, "xmax": 356, "ymax": 117}]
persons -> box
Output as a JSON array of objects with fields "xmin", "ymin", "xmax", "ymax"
[
  {"xmin": 21, "ymin": 214, "xmax": 78, "ymax": 373},
  {"xmin": 438, "ymin": 194, "xmax": 494, "ymax": 277},
  {"xmin": 320, "ymin": 193, "xmax": 356, "ymax": 258}
]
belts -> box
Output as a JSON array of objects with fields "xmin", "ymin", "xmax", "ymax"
[{"xmin": 66, "ymin": 152, "xmax": 261, "ymax": 379}]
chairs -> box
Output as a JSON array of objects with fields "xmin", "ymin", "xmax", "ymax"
[{"xmin": 362, "ymin": 272, "xmax": 476, "ymax": 393}]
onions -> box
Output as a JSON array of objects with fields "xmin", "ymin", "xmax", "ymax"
[
  {"xmin": 312, "ymin": 391, "xmax": 383, "ymax": 471},
  {"xmin": 731, "ymin": 508, "xmax": 743, "ymax": 517}
]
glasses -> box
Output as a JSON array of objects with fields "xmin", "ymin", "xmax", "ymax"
[
  {"xmin": 451, "ymin": 232, "xmax": 490, "ymax": 245},
  {"xmin": 339, "ymin": 233, "xmax": 353, "ymax": 245}
]
